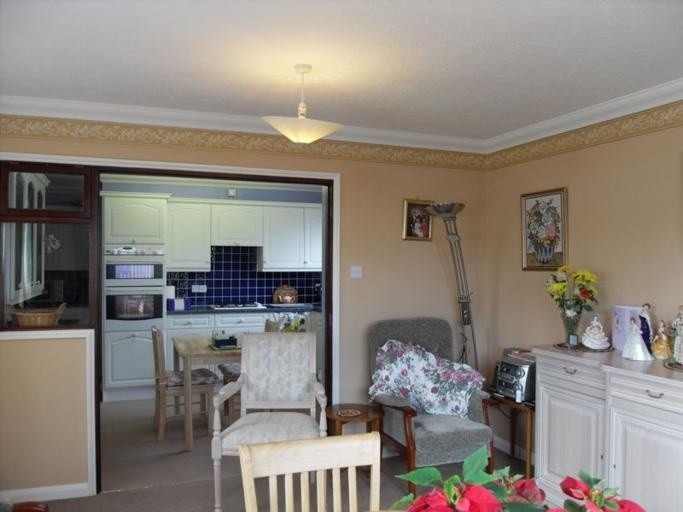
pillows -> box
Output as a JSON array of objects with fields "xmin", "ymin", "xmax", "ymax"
[{"xmin": 368, "ymin": 339, "xmax": 485, "ymax": 419}]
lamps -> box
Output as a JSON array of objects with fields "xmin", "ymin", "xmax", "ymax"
[
  {"xmin": 427, "ymin": 201, "xmax": 478, "ymax": 370},
  {"xmin": 262, "ymin": 114, "xmax": 344, "ymax": 145}
]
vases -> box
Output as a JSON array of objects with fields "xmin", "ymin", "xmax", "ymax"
[{"xmin": 559, "ymin": 312, "xmax": 583, "ymax": 347}]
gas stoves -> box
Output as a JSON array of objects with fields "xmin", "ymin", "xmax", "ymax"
[{"xmin": 209, "ymin": 302, "xmax": 267, "ymax": 310}]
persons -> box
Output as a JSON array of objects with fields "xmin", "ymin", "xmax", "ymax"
[
  {"xmin": 408, "ymin": 212, "xmax": 428, "ymax": 238},
  {"xmin": 670, "ymin": 305, "xmax": 683, "ymax": 365},
  {"xmin": 652, "ymin": 320, "xmax": 672, "ymax": 360},
  {"xmin": 637, "ymin": 302, "xmax": 653, "ymax": 356},
  {"xmin": 622, "ymin": 317, "xmax": 655, "ymax": 362},
  {"xmin": 582, "ymin": 312, "xmax": 610, "ymax": 350}
]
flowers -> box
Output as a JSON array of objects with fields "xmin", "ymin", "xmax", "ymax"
[
  {"xmin": 390, "ymin": 445, "xmax": 648, "ymax": 512},
  {"xmin": 544, "ymin": 265, "xmax": 601, "ymax": 312}
]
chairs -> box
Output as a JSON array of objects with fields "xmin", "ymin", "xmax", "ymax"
[
  {"xmin": 238, "ymin": 430, "xmax": 380, "ymax": 512},
  {"xmin": 366, "ymin": 316, "xmax": 495, "ymax": 497},
  {"xmin": 211, "ymin": 331, "xmax": 327, "ymax": 511},
  {"xmin": 217, "ymin": 359, "xmax": 274, "ymax": 426},
  {"xmin": 151, "ymin": 324, "xmax": 219, "ymax": 442}
]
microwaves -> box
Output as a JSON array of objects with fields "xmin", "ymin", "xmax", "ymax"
[{"xmin": 30, "ymin": 280, "xmax": 64, "ymax": 303}]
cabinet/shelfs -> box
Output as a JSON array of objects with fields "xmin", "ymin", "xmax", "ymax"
[
  {"xmin": 3, "ymin": 172, "xmax": 51, "ymax": 305},
  {"xmin": 531, "ymin": 343, "xmax": 604, "ymax": 509},
  {"xmin": 211, "ymin": 205, "xmax": 263, "ymax": 246},
  {"xmin": 104, "ymin": 197, "xmax": 167, "ymax": 257},
  {"xmin": 261, "ymin": 208, "xmax": 323, "ymax": 270},
  {"xmin": 602, "ymin": 350, "xmax": 683, "ymax": 511},
  {"xmin": 106, "ymin": 332, "xmax": 164, "ymax": 388},
  {"xmin": 166, "ymin": 315, "xmax": 215, "ymax": 371},
  {"xmin": 216, "ymin": 316, "xmax": 265, "ymax": 337},
  {"xmin": 165, "ymin": 202, "xmax": 211, "ymax": 273}
]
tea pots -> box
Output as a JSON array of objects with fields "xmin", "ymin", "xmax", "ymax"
[{"xmin": 272, "ymin": 285, "xmax": 299, "ymax": 305}]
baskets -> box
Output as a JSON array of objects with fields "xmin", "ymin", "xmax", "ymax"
[{"xmin": 7, "ymin": 302, "xmax": 67, "ymax": 328}]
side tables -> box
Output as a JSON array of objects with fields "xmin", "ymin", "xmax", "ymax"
[{"xmin": 325, "ymin": 404, "xmax": 386, "ymax": 478}]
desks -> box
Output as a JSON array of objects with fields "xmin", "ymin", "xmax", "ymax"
[
  {"xmin": 482, "ymin": 397, "xmax": 536, "ymax": 479},
  {"xmin": 172, "ymin": 332, "xmax": 242, "ymax": 452}
]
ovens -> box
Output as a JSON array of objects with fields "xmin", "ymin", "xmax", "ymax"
[
  {"xmin": 101, "ymin": 255, "xmax": 166, "ymax": 287},
  {"xmin": 103, "ymin": 288, "xmax": 166, "ymax": 332}
]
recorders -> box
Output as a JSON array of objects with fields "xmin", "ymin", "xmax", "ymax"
[{"xmin": 496, "ymin": 353, "xmax": 536, "ymax": 403}]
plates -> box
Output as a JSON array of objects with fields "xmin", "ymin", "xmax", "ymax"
[{"xmin": 269, "ymin": 304, "xmax": 306, "ymax": 307}]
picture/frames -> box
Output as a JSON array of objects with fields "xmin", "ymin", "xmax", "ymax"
[
  {"xmin": 401, "ymin": 198, "xmax": 433, "ymax": 242},
  {"xmin": 519, "ymin": 187, "xmax": 569, "ymax": 273}
]
